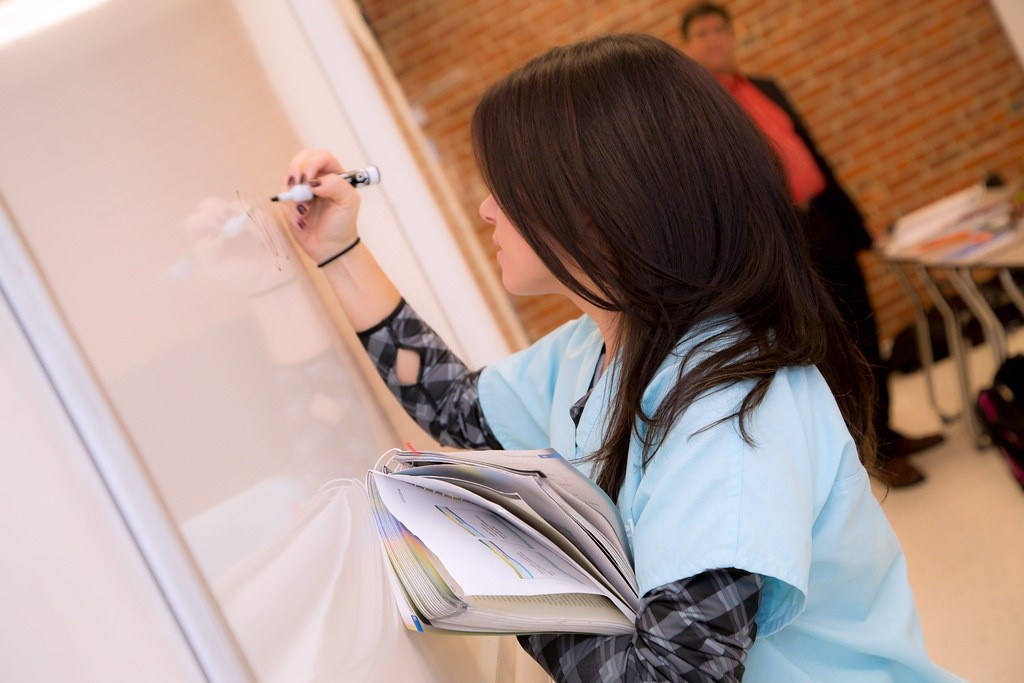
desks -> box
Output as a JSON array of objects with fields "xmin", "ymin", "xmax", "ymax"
[{"xmin": 875, "ymin": 176, "xmax": 1024, "ymax": 450}]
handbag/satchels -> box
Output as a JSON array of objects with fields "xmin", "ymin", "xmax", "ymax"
[{"xmin": 975, "ymin": 354, "xmax": 1024, "ymax": 496}]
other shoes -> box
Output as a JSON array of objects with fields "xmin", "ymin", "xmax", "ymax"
[
  {"xmin": 890, "ymin": 428, "xmax": 946, "ymax": 457},
  {"xmin": 880, "ymin": 459, "xmax": 923, "ymax": 487}
]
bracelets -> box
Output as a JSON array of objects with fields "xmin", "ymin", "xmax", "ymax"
[{"xmin": 318, "ymin": 238, "xmax": 360, "ymax": 267}]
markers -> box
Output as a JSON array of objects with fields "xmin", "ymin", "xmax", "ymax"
[{"xmin": 269, "ymin": 163, "xmax": 383, "ymax": 203}]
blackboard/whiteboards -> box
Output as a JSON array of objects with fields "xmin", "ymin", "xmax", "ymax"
[{"xmin": 2, "ymin": 1, "xmax": 518, "ymax": 683}]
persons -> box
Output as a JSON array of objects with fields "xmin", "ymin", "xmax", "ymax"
[
  {"xmin": 281, "ymin": 33, "xmax": 968, "ymax": 683},
  {"xmin": 681, "ymin": 5, "xmax": 945, "ymax": 487}
]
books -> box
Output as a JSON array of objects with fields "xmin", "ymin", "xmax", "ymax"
[
  {"xmin": 365, "ymin": 448, "xmax": 638, "ymax": 633},
  {"xmin": 914, "ymin": 231, "xmax": 1014, "ymax": 266}
]
